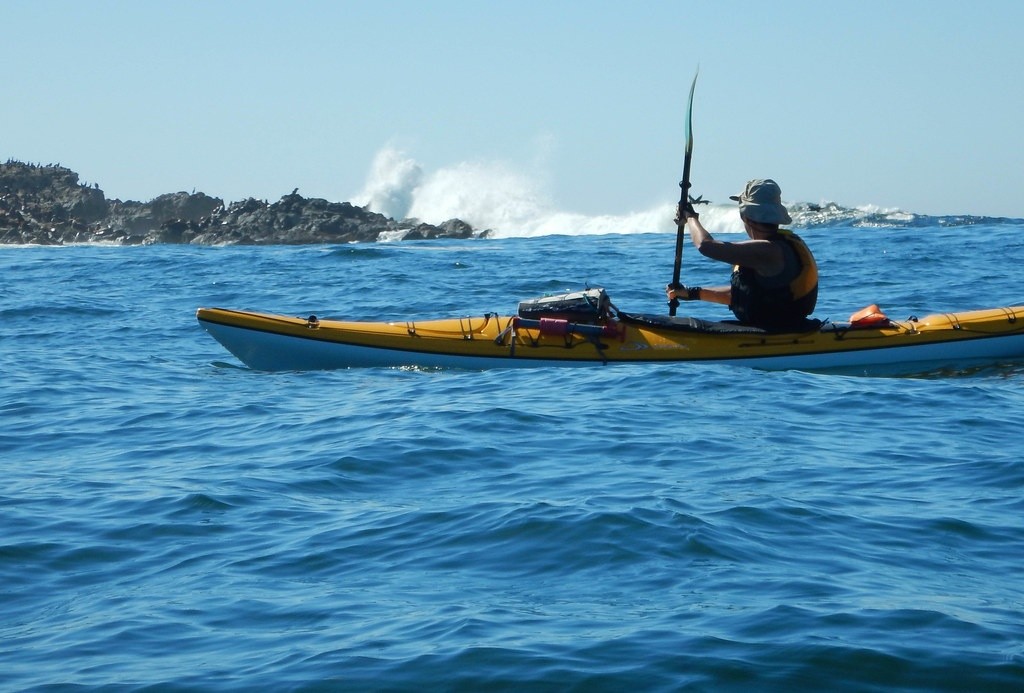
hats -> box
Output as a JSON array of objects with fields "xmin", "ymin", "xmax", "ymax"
[{"xmin": 729, "ymin": 179, "xmax": 792, "ymax": 225}]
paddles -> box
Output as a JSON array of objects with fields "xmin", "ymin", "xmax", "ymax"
[{"xmin": 667, "ymin": 76, "xmax": 698, "ymax": 316}]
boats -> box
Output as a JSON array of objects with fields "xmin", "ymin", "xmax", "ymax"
[{"xmin": 195, "ymin": 288, "xmax": 1024, "ymax": 371}]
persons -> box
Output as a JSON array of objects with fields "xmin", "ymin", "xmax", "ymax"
[{"xmin": 665, "ymin": 178, "xmax": 819, "ymax": 327}]
